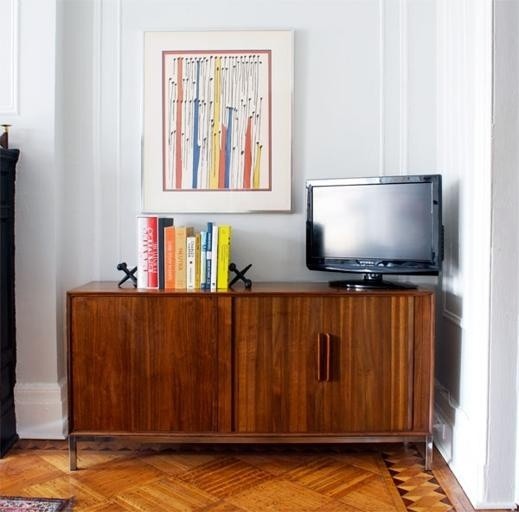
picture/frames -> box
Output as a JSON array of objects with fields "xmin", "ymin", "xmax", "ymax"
[{"xmin": 139, "ymin": 29, "xmax": 296, "ymax": 216}]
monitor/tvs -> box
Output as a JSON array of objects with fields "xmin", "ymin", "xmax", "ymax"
[{"xmin": 305, "ymin": 174, "xmax": 444, "ymax": 291}]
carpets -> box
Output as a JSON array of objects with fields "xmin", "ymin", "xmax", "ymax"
[{"xmin": 0, "ymin": 493, "xmax": 78, "ymax": 511}]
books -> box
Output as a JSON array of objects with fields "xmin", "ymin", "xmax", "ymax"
[{"xmin": 135, "ymin": 215, "xmax": 233, "ymax": 291}]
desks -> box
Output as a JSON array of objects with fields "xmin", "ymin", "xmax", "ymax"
[{"xmin": 231, "ymin": 295, "xmax": 415, "ymax": 433}]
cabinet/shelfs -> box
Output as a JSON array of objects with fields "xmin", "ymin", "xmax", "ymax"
[
  {"xmin": 0, "ymin": 146, "xmax": 25, "ymax": 457},
  {"xmin": 69, "ymin": 295, "xmax": 220, "ymax": 434}
]
